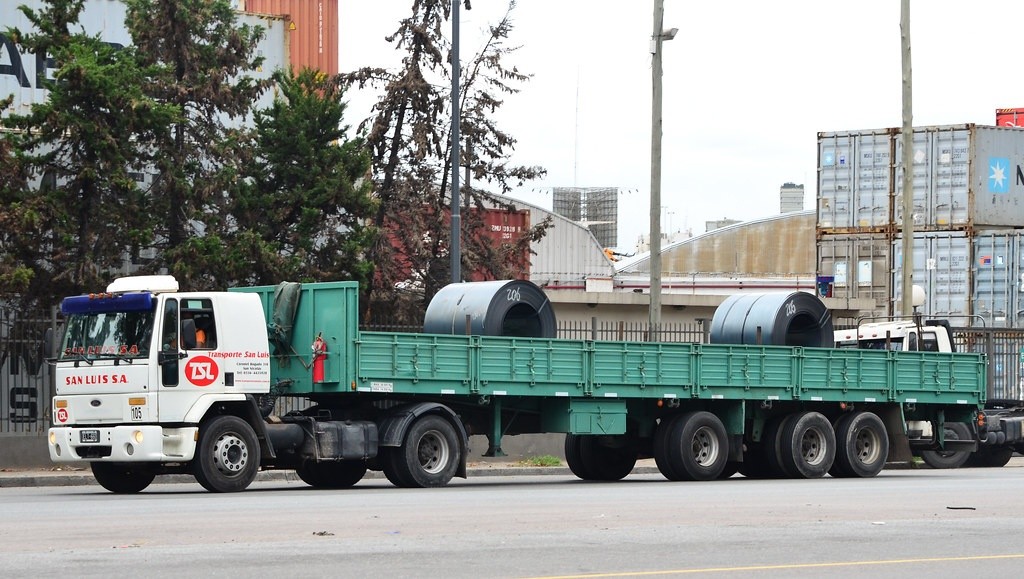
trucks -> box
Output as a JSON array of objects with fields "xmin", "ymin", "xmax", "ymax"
[{"xmin": 829, "ymin": 307, "xmax": 1024, "ymax": 467}]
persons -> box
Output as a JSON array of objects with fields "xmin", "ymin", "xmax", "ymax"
[{"xmin": 171, "ymin": 312, "xmax": 206, "ymax": 351}]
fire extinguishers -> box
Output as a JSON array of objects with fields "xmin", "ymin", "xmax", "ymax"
[{"xmin": 311, "ymin": 332, "xmax": 327, "ymax": 381}]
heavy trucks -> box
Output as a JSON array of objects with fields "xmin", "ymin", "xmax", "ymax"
[{"xmin": 50, "ymin": 273, "xmax": 989, "ymax": 491}]
